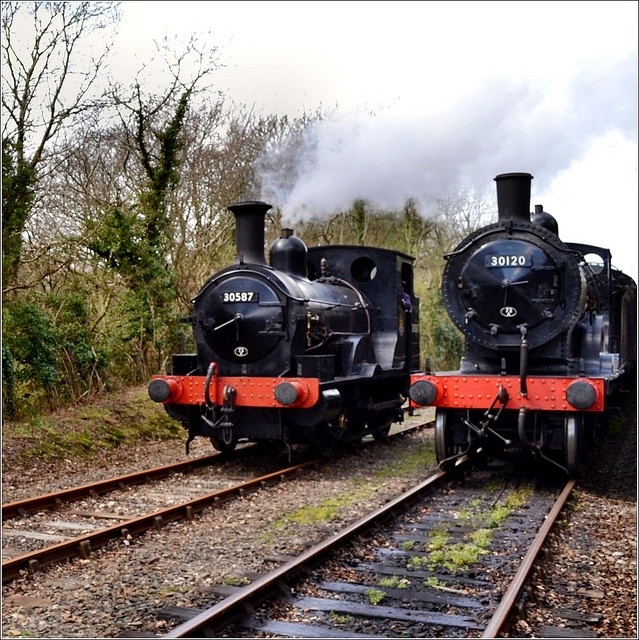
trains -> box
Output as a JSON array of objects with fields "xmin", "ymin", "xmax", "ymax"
[
  {"xmin": 148, "ymin": 201, "xmax": 419, "ymax": 463},
  {"xmin": 409, "ymin": 171, "xmax": 637, "ymax": 481}
]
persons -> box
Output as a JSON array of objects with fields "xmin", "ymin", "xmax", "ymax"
[{"xmin": 398, "ymin": 280, "xmax": 413, "ymax": 337}]
